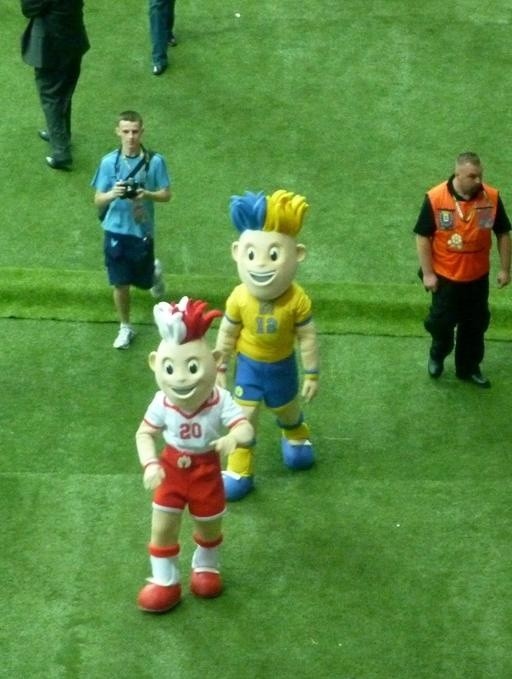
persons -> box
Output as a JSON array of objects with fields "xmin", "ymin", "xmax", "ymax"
[
  {"xmin": 148, "ymin": 0, "xmax": 177, "ymax": 76},
  {"xmin": 16, "ymin": 0, "xmax": 92, "ymax": 169},
  {"xmin": 90, "ymin": 112, "xmax": 164, "ymax": 348},
  {"xmin": 412, "ymin": 152, "xmax": 512, "ymax": 386}
]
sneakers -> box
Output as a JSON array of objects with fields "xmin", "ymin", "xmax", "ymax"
[
  {"xmin": 114, "ymin": 324, "xmax": 138, "ymax": 349},
  {"xmin": 150, "ymin": 259, "xmax": 165, "ymax": 298}
]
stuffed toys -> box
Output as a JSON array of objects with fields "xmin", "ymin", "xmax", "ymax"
[
  {"xmin": 214, "ymin": 188, "xmax": 321, "ymax": 501},
  {"xmin": 130, "ymin": 296, "xmax": 255, "ymax": 615}
]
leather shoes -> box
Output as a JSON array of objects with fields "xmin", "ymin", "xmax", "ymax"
[
  {"xmin": 39, "ymin": 130, "xmax": 50, "ymax": 141},
  {"xmin": 168, "ymin": 36, "xmax": 177, "ymax": 47},
  {"xmin": 428, "ymin": 355, "xmax": 443, "ymax": 378},
  {"xmin": 46, "ymin": 155, "xmax": 73, "ymax": 168},
  {"xmin": 152, "ymin": 65, "xmax": 166, "ymax": 75},
  {"xmin": 455, "ymin": 367, "xmax": 490, "ymax": 387}
]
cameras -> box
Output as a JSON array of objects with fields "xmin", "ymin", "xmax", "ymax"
[{"xmin": 118, "ymin": 179, "xmax": 145, "ymax": 200}]
